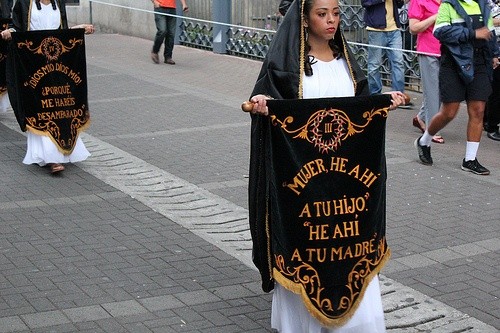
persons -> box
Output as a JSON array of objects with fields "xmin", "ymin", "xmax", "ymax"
[
  {"xmin": 407, "ymin": 0, "xmax": 500, "ymax": 175},
  {"xmin": 0, "ymin": 0, "xmax": 22, "ymax": 91},
  {"xmin": 151, "ymin": 0, "xmax": 189, "ymax": 65},
  {"xmin": 249, "ymin": 0, "xmax": 407, "ymax": 333},
  {"xmin": 1, "ymin": 0, "xmax": 94, "ymax": 174},
  {"xmin": 361, "ymin": 0, "xmax": 414, "ymax": 108}
]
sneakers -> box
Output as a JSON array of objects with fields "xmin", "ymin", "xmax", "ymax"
[
  {"xmin": 414, "ymin": 134, "xmax": 433, "ymax": 164},
  {"xmin": 461, "ymin": 157, "xmax": 490, "ymax": 174}
]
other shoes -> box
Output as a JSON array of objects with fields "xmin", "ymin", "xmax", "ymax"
[
  {"xmin": 432, "ymin": 134, "xmax": 445, "ymax": 144},
  {"xmin": 151, "ymin": 50, "xmax": 159, "ymax": 63},
  {"xmin": 413, "ymin": 115, "xmax": 427, "ymax": 133},
  {"xmin": 164, "ymin": 55, "xmax": 175, "ymax": 63},
  {"xmin": 487, "ymin": 131, "xmax": 500, "ymax": 140},
  {"xmin": 51, "ymin": 163, "xmax": 65, "ymax": 172},
  {"xmin": 398, "ymin": 101, "xmax": 413, "ymax": 108}
]
